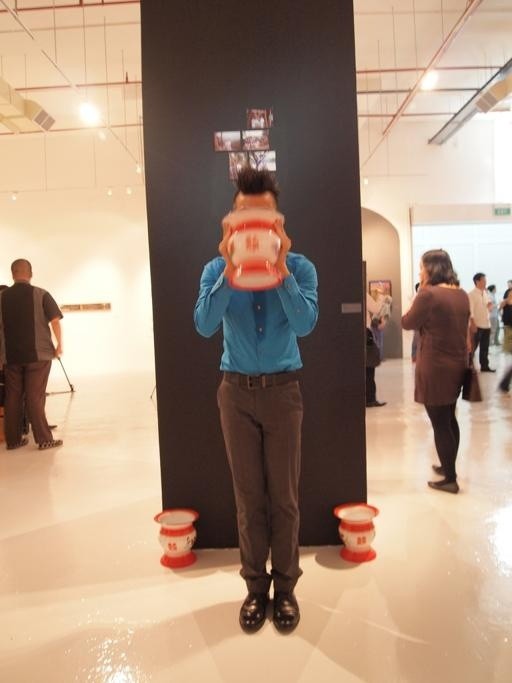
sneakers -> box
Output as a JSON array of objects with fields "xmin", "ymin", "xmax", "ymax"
[
  {"xmin": 7, "ymin": 438, "xmax": 27, "ymax": 450},
  {"xmin": 39, "ymin": 440, "xmax": 63, "ymax": 449}
]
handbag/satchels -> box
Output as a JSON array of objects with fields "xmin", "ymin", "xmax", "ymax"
[{"xmin": 462, "ymin": 366, "xmax": 483, "ymax": 402}]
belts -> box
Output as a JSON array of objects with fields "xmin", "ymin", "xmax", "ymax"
[{"xmin": 223, "ymin": 370, "xmax": 305, "ymax": 390}]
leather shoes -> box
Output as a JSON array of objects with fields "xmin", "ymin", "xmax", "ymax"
[
  {"xmin": 430, "ymin": 479, "xmax": 459, "ymax": 494},
  {"xmin": 365, "ymin": 400, "xmax": 385, "ymax": 408},
  {"xmin": 433, "ymin": 464, "xmax": 456, "ymax": 476},
  {"xmin": 480, "ymin": 366, "xmax": 495, "ymax": 372}
]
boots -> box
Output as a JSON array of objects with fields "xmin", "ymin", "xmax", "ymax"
[
  {"xmin": 272, "ymin": 570, "xmax": 304, "ymax": 631},
  {"xmin": 239, "ymin": 575, "xmax": 271, "ymax": 633}
]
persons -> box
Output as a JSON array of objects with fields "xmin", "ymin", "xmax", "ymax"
[
  {"xmin": 366, "ymin": 292, "xmax": 392, "ymax": 407},
  {"xmin": 409, "ymin": 273, "xmax": 511, "ymax": 393},
  {"xmin": 194, "ymin": 166, "xmax": 320, "ymax": 633},
  {"xmin": 0, "ymin": 259, "xmax": 64, "ymax": 449},
  {"xmin": 397, "ymin": 249, "xmax": 482, "ymax": 492}
]
